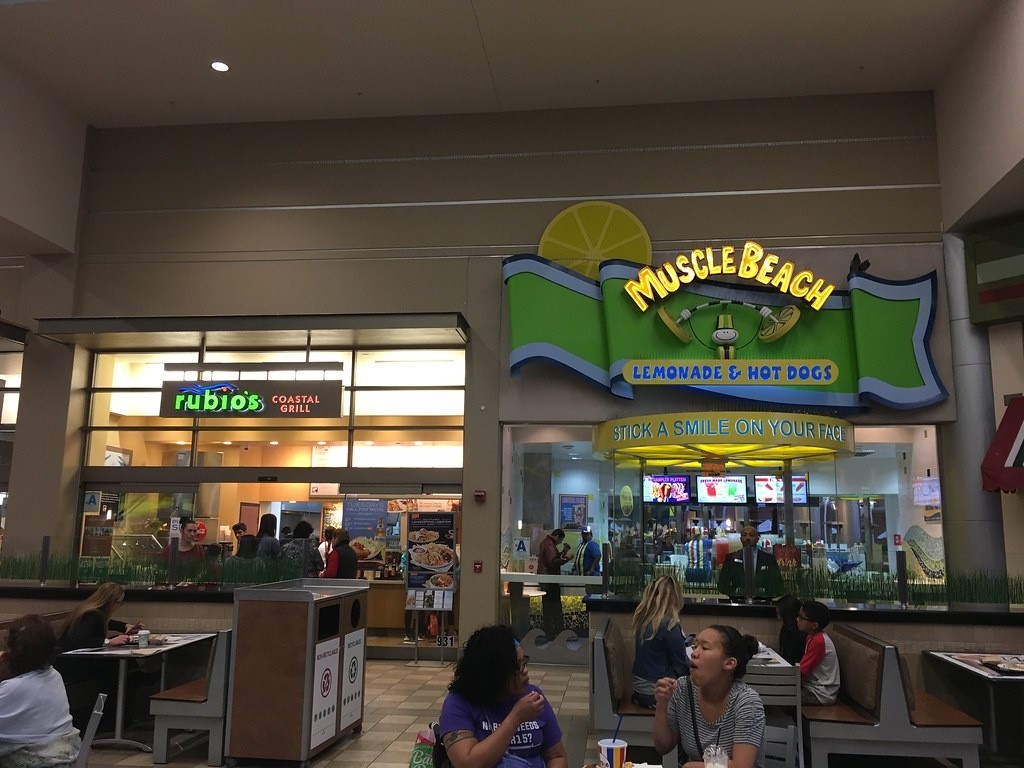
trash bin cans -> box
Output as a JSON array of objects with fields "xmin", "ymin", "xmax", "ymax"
[{"xmin": 224, "ymin": 578, "xmax": 370, "ymax": 768}]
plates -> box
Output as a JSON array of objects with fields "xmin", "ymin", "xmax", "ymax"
[
  {"xmin": 997, "ymin": 664, "xmax": 1024, "ymax": 671},
  {"xmin": 409, "ymin": 531, "xmax": 439, "ymax": 543},
  {"xmin": 412, "ymin": 547, "xmax": 426, "ymax": 555},
  {"xmin": 411, "ymin": 544, "xmax": 454, "ymax": 568}
]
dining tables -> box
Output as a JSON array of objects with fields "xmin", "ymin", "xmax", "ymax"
[
  {"xmin": 681, "ymin": 629, "xmax": 795, "ymax": 761},
  {"xmin": 922, "ymin": 647, "xmax": 1024, "ymax": 751},
  {"xmin": 62, "ymin": 630, "xmax": 217, "ymax": 753}
]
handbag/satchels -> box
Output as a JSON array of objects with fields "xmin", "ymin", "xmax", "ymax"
[
  {"xmin": 409, "ymin": 721, "xmax": 438, "ymax": 768},
  {"xmin": 429, "ymin": 615, "xmax": 441, "ymax": 637}
]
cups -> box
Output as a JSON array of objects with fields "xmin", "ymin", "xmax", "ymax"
[
  {"xmin": 138, "ymin": 629, "xmax": 150, "ymax": 647},
  {"xmin": 702, "ymin": 744, "xmax": 728, "ymax": 768},
  {"xmin": 598, "ymin": 738, "xmax": 628, "ymax": 768}
]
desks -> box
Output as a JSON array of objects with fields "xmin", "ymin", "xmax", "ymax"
[{"xmin": 501, "ymin": 590, "xmax": 546, "ymax": 639}]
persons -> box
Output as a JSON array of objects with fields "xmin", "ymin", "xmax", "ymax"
[
  {"xmin": 440, "ymin": 625, "xmax": 568, "ymax": 768},
  {"xmin": 609, "ymin": 526, "xmax": 714, "ymax": 587},
  {"xmin": 654, "ymin": 625, "xmax": 766, "ymax": 768},
  {"xmin": 0, "ymin": 615, "xmax": 81, "ymax": 768},
  {"xmin": 58, "ymin": 582, "xmax": 145, "ymax": 728},
  {"xmin": 568, "ymin": 529, "xmax": 602, "ymax": 576},
  {"xmin": 776, "ymin": 595, "xmax": 808, "ymax": 665},
  {"xmin": 539, "ymin": 528, "xmax": 574, "ymax": 638},
  {"xmin": 717, "ymin": 526, "xmax": 785, "ymax": 596},
  {"xmin": 157, "ymin": 514, "xmax": 358, "ymax": 578},
  {"xmin": 775, "ymin": 601, "xmax": 840, "ymax": 725},
  {"xmin": 633, "ymin": 575, "xmax": 696, "ymax": 709}
]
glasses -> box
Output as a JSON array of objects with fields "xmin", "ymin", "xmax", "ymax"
[
  {"xmin": 515, "ymin": 654, "xmax": 530, "ymax": 673},
  {"xmin": 798, "ymin": 613, "xmax": 812, "ymax": 623}
]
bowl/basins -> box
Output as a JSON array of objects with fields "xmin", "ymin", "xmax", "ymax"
[{"xmin": 431, "ymin": 574, "xmax": 453, "ymax": 589}]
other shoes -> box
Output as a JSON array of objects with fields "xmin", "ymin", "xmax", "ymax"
[
  {"xmin": 418, "ymin": 635, "xmax": 429, "ymax": 641},
  {"xmin": 404, "ymin": 634, "xmax": 412, "ymax": 641}
]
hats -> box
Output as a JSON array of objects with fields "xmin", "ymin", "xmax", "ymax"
[
  {"xmin": 581, "ymin": 526, "xmax": 593, "ymax": 535},
  {"xmin": 699, "ymin": 526, "xmax": 708, "ymax": 533},
  {"xmin": 691, "ymin": 526, "xmax": 702, "ymax": 536}
]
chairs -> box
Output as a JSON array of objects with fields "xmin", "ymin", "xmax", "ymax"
[
  {"xmin": 74, "ymin": 693, "xmax": 106, "ymax": 768},
  {"xmin": 763, "ymin": 724, "xmax": 804, "ymax": 768}
]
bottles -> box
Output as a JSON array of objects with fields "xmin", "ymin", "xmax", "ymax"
[
  {"xmin": 384, "ymin": 559, "xmax": 388, "ymax": 577},
  {"xmin": 374, "ymin": 518, "xmax": 386, "ymax": 548},
  {"xmin": 391, "ymin": 558, "xmax": 396, "ymax": 577}
]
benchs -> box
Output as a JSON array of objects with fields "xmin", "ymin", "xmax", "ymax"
[
  {"xmin": 150, "ymin": 628, "xmax": 232, "ymax": 767},
  {"xmin": 800, "ymin": 622, "xmax": 984, "ymax": 768},
  {"xmin": 0, "ymin": 608, "xmax": 107, "ymax": 734},
  {"xmin": 591, "ymin": 617, "xmax": 677, "ymax": 768}
]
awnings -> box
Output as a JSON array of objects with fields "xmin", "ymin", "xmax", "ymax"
[{"xmin": 981, "ymin": 397, "xmax": 1024, "ymax": 492}]
